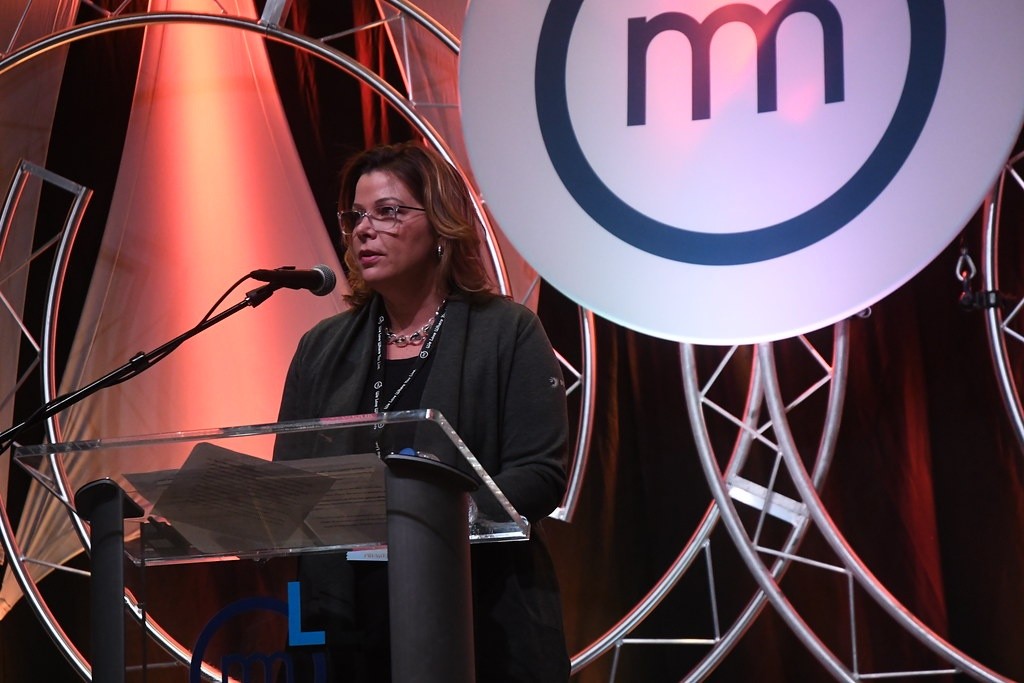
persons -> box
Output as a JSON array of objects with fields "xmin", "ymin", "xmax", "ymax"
[{"xmin": 271, "ymin": 138, "xmax": 570, "ymax": 683}]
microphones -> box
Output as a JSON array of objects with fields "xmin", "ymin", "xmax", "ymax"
[{"xmin": 250, "ymin": 264, "xmax": 337, "ymax": 296}]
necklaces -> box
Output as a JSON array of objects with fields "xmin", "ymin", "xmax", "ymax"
[{"xmin": 383, "ymin": 296, "xmax": 447, "ymax": 347}]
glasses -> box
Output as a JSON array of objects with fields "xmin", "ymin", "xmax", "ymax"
[{"xmin": 337, "ymin": 204, "xmax": 425, "ymax": 235}]
